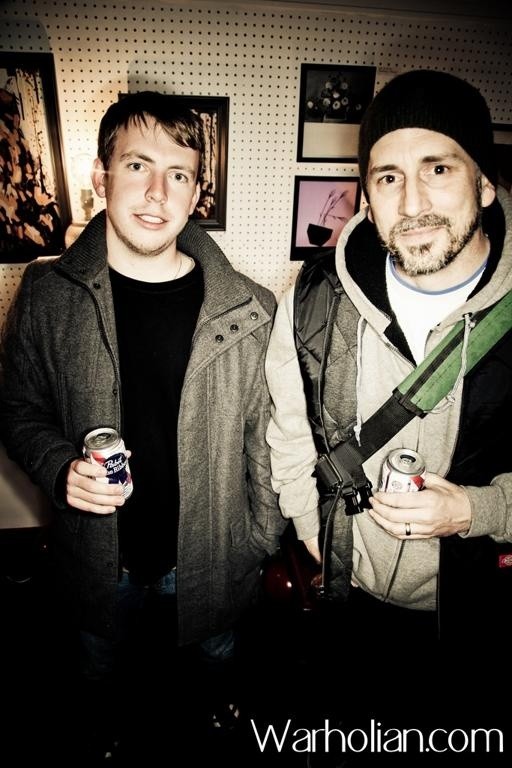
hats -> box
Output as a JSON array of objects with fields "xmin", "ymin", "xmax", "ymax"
[{"xmin": 357, "ymin": 69, "xmax": 499, "ymax": 203}]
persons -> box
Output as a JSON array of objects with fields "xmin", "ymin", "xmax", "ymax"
[
  {"xmin": 262, "ymin": 71, "xmax": 512, "ymax": 767},
  {"xmin": 0, "ymin": 91, "xmax": 289, "ymax": 768}
]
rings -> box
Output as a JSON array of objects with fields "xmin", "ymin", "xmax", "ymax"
[{"xmin": 406, "ymin": 522, "xmax": 412, "ymax": 536}]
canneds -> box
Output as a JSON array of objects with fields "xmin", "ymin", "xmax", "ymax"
[
  {"xmin": 83, "ymin": 425, "xmax": 134, "ymax": 506},
  {"xmin": 377, "ymin": 448, "xmax": 425, "ymax": 499}
]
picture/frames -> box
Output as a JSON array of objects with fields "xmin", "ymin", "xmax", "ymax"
[{"xmin": 0, "ymin": 51, "xmax": 377, "ymax": 265}]
